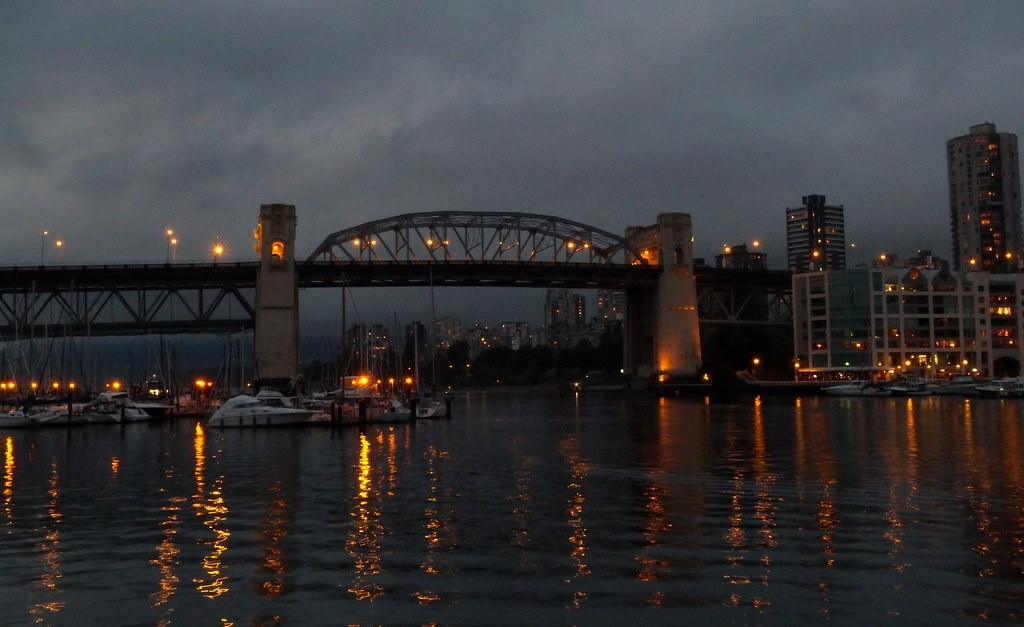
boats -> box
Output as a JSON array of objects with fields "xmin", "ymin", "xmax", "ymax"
[
  {"xmin": 30, "ymin": 390, "xmax": 152, "ymax": 426},
  {"xmin": 0, "ymin": 408, "xmax": 39, "ymax": 428},
  {"xmin": 206, "ymin": 395, "xmax": 325, "ymax": 427},
  {"xmin": 883, "ymin": 377, "xmax": 933, "ymax": 397},
  {"xmin": 926, "ymin": 375, "xmax": 983, "ymax": 397},
  {"xmin": 820, "ymin": 379, "xmax": 891, "ymax": 397},
  {"xmin": 100, "ymin": 391, "xmax": 176, "ymax": 417},
  {"xmin": 975, "ymin": 377, "xmax": 1024, "ymax": 399}
]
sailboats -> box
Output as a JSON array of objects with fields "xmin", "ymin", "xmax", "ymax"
[{"xmin": 306, "ymin": 316, "xmax": 447, "ymax": 423}]
